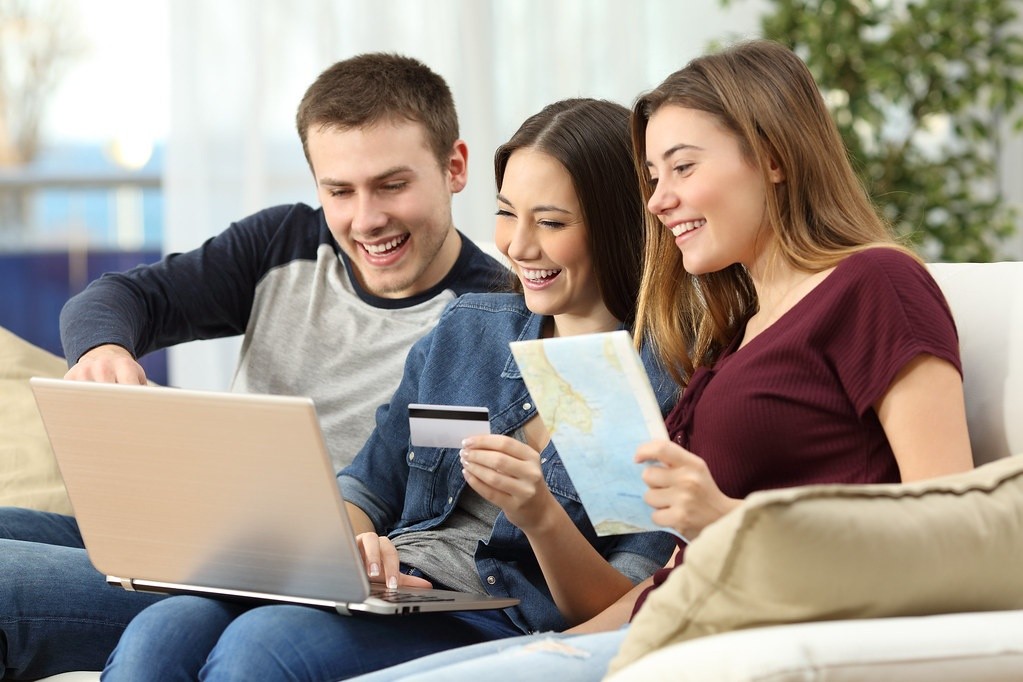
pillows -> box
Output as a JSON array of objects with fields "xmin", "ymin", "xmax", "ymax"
[{"xmin": 608, "ymin": 453, "xmax": 1023, "ymax": 676}]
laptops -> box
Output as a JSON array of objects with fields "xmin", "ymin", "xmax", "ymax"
[{"xmin": 27, "ymin": 377, "xmax": 521, "ymax": 616}]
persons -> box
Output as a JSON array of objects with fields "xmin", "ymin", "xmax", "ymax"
[
  {"xmin": 0, "ymin": 54, "xmax": 518, "ymax": 682},
  {"xmin": 340, "ymin": 39, "xmax": 975, "ymax": 682},
  {"xmin": 99, "ymin": 96, "xmax": 680, "ymax": 682}
]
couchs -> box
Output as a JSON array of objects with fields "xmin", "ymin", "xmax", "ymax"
[{"xmin": 602, "ymin": 258, "xmax": 1023, "ymax": 682}]
